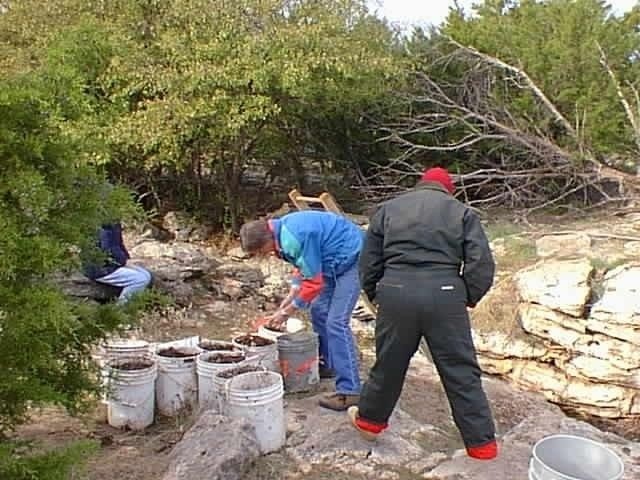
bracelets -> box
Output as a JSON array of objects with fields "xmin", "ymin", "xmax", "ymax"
[{"xmin": 281, "ymin": 309, "xmax": 290, "ymax": 318}]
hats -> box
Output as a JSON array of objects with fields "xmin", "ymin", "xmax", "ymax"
[{"xmin": 423, "ymin": 167, "xmax": 456, "ymax": 194}]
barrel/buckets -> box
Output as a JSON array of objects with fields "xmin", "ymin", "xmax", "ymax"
[
  {"xmin": 528, "ymin": 434, "xmax": 626, "ymax": 480},
  {"xmin": 97, "ymin": 318, "xmax": 322, "ymax": 455}
]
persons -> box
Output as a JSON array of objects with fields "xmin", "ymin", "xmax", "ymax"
[
  {"xmin": 238, "ymin": 209, "xmax": 367, "ymax": 411},
  {"xmin": 78, "ymin": 181, "xmax": 153, "ymax": 305},
  {"xmin": 346, "ymin": 164, "xmax": 498, "ymax": 461}
]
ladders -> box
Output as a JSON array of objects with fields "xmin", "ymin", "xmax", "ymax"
[{"xmin": 288, "ymin": 189, "xmax": 434, "ymax": 364}]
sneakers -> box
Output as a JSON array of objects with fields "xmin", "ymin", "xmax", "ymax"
[
  {"xmin": 348, "ymin": 405, "xmax": 378, "ymax": 442},
  {"xmin": 321, "ymin": 366, "xmax": 336, "ymax": 378},
  {"xmin": 319, "ymin": 392, "xmax": 361, "ymax": 411}
]
